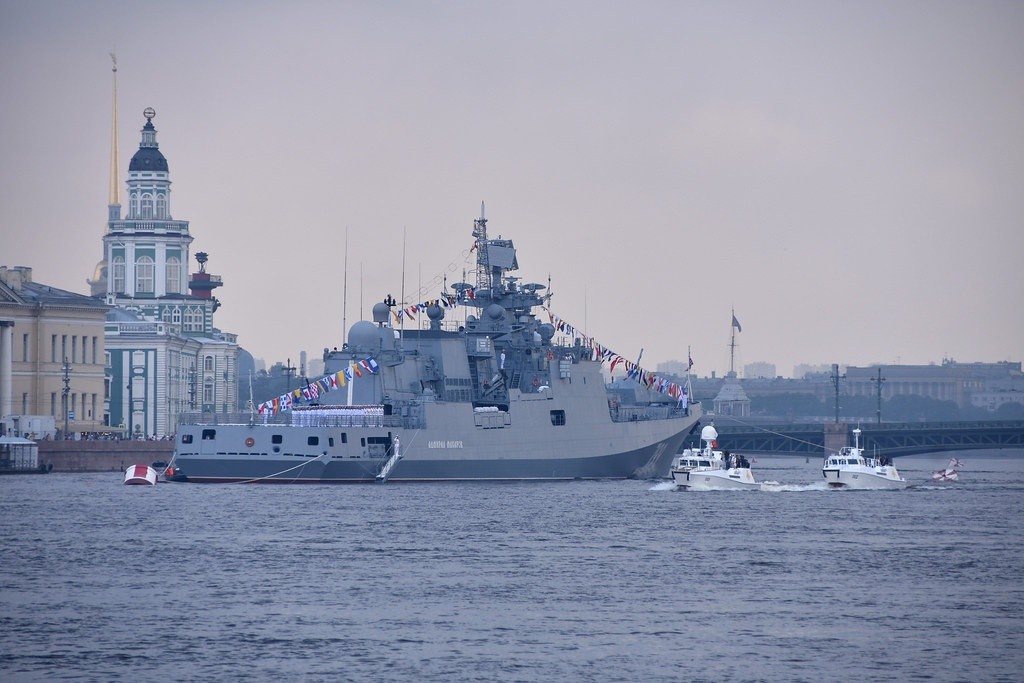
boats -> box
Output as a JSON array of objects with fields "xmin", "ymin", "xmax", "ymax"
[
  {"xmin": 672, "ymin": 426, "xmax": 761, "ymax": 489},
  {"xmin": 823, "ymin": 427, "xmax": 907, "ymax": 489},
  {"xmin": 166, "ymin": 201, "xmax": 701, "ymax": 483}
]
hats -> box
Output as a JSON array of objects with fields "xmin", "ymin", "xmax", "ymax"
[{"xmin": 292, "ymin": 404, "xmax": 384, "ymax": 409}]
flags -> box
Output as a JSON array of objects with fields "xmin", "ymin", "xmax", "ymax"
[
  {"xmin": 344, "ymin": 368, "xmax": 352, "ymax": 381},
  {"xmin": 359, "ymin": 360, "xmax": 373, "ymax": 374},
  {"xmin": 352, "ymin": 364, "xmax": 362, "ymax": 377},
  {"xmin": 330, "ymin": 374, "xmax": 339, "ymax": 389},
  {"xmin": 732, "ymin": 316, "xmax": 742, "ymax": 332},
  {"xmin": 685, "ymin": 357, "xmax": 694, "ymax": 371},
  {"xmin": 542, "ymin": 307, "xmax": 683, "ymax": 400},
  {"xmin": 337, "ymin": 371, "xmax": 346, "ymax": 386},
  {"xmin": 366, "ymin": 356, "xmax": 379, "ymax": 372},
  {"xmin": 392, "ymin": 287, "xmax": 478, "ymax": 324},
  {"xmin": 258, "ymin": 375, "xmax": 329, "ymax": 415}
]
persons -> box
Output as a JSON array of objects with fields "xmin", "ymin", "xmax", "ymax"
[
  {"xmin": 92, "ymin": 432, "xmax": 119, "ymax": 440},
  {"xmin": 151, "ymin": 433, "xmax": 175, "ymax": 441},
  {"xmin": 292, "ymin": 404, "xmax": 384, "ymax": 427},
  {"xmin": 880, "ymin": 456, "xmax": 887, "ymax": 466},
  {"xmin": 736, "ymin": 455, "xmax": 750, "ymax": 468},
  {"xmin": 725, "ymin": 451, "xmax": 730, "ymax": 469}
]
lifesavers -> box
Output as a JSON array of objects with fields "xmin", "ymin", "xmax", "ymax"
[{"xmin": 245, "ymin": 437, "xmax": 254, "ymax": 447}]
